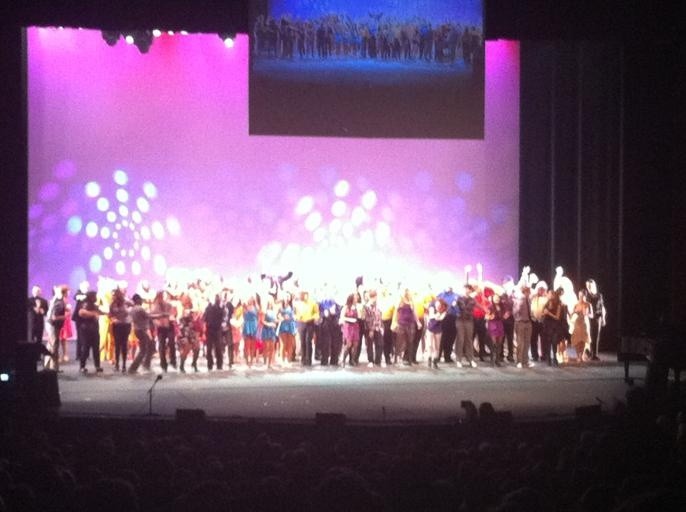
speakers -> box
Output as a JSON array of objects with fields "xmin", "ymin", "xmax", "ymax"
[
  {"xmin": 575, "ymin": 405, "xmax": 601, "ymax": 417},
  {"xmin": 316, "ymin": 412, "xmax": 346, "ymax": 424},
  {"xmin": 177, "ymin": 408, "xmax": 205, "ymax": 418}
]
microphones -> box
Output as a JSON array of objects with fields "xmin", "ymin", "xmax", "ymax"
[{"xmin": 154, "ymin": 374, "xmax": 162, "ymax": 384}]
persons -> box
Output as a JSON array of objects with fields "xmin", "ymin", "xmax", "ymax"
[{"xmin": 250, "ymin": 13, "xmax": 483, "ymax": 72}]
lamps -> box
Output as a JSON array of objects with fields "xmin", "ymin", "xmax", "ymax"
[{"xmin": 97, "ymin": 25, "xmax": 238, "ymax": 56}]
